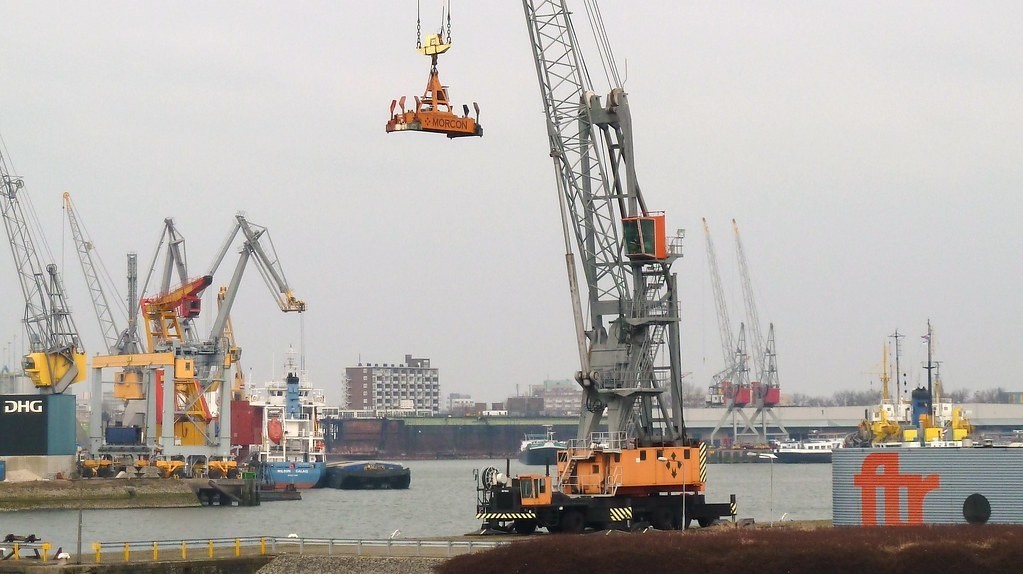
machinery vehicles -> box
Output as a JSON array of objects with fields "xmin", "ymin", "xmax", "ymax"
[{"xmin": 387, "ymin": 0, "xmax": 738, "ymax": 537}]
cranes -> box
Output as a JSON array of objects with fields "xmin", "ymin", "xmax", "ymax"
[{"xmin": 706, "ymin": 217, "xmax": 781, "ymax": 407}]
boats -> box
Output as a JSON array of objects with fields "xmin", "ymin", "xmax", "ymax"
[
  {"xmin": 520, "ymin": 424, "xmax": 569, "ymax": 464},
  {"xmin": 0, "ymin": 132, "xmax": 411, "ymax": 490},
  {"xmin": 773, "ymin": 318, "xmax": 982, "ymax": 463}
]
